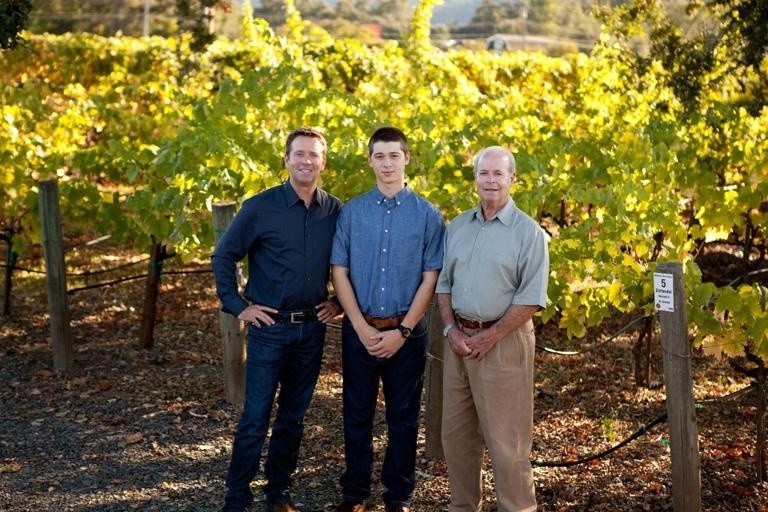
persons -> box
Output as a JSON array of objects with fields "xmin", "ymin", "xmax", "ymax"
[
  {"xmin": 432, "ymin": 144, "xmax": 555, "ymax": 512},
  {"xmin": 209, "ymin": 128, "xmax": 346, "ymax": 511},
  {"xmin": 327, "ymin": 125, "xmax": 447, "ymax": 511}
]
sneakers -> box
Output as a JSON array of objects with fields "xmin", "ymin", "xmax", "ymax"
[
  {"xmin": 218, "ymin": 491, "xmax": 254, "ymax": 512},
  {"xmin": 265, "ymin": 491, "xmax": 301, "ymax": 512},
  {"xmin": 335, "ymin": 497, "xmax": 366, "ymax": 512},
  {"xmin": 383, "ymin": 502, "xmax": 411, "ymax": 512}
]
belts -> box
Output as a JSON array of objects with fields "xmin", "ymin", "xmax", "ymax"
[
  {"xmin": 260, "ymin": 310, "xmax": 324, "ymax": 324},
  {"xmin": 452, "ymin": 313, "xmax": 501, "ymax": 331},
  {"xmin": 364, "ymin": 313, "xmax": 408, "ymax": 330}
]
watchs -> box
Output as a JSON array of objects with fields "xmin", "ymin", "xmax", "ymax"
[
  {"xmin": 396, "ymin": 324, "xmax": 415, "ymax": 341},
  {"xmin": 442, "ymin": 322, "xmax": 456, "ymax": 338}
]
